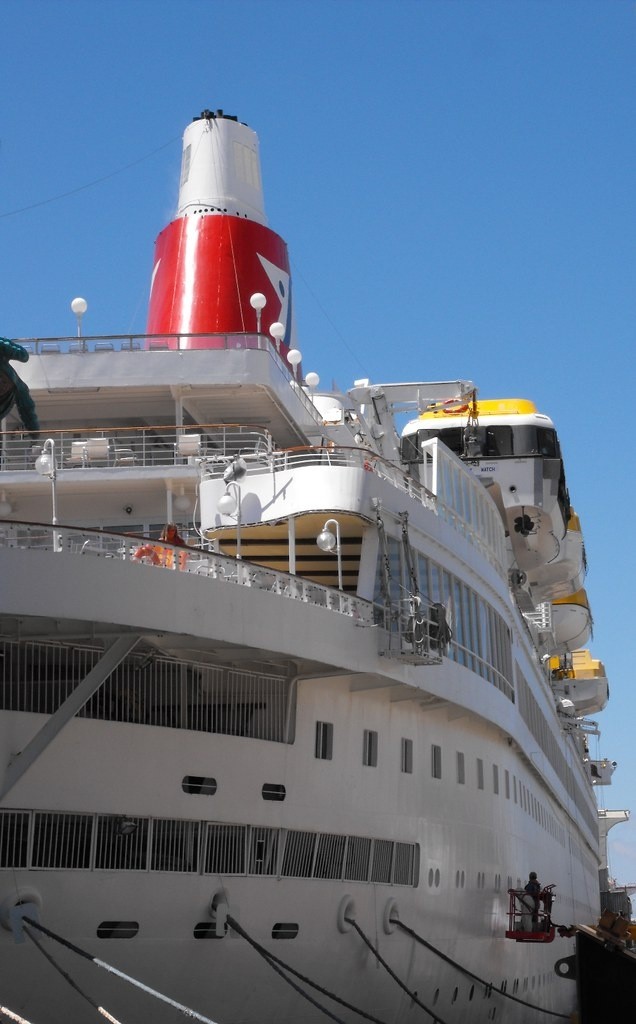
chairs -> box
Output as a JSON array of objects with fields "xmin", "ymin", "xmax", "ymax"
[
  {"xmin": 179, "ymin": 434, "xmax": 202, "ymax": 463},
  {"xmin": 66, "ymin": 440, "xmax": 138, "ymax": 469},
  {"xmin": 22, "ymin": 342, "xmax": 168, "ymax": 354}
]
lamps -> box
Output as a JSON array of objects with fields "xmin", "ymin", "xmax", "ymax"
[
  {"xmin": 71, "ymin": 298, "xmax": 88, "ymax": 353},
  {"xmin": 217, "ymin": 481, "xmax": 241, "ymax": 559},
  {"xmin": 119, "ymin": 818, "xmax": 139, "ymax": 835},
  {"xmin": 270, "ymin": 322, "xmax": 285, "ymax": 353},
  {"xmin": 223, "ymin": 456, "xmax": 247, "ymax": 483},
  {"xmin": 287, "ymin": 350, "xmax": 302, "ymax": 381},
  {"xmin": 316, "ymin": 519, "xmax": 342, "ymax": 591},
  {"xmin": 35, "ymin": 438, "xmax": 58, "ymax": 552},
  {"xmin": 250, "ymin": 293, "xmax": 267, "ymax": 349},
  {"xmin": 306, "ymin": 372, "xmax": 319, "ymax": 404},
  {"xmin": 175, "ymin": 495, "xmax": 191, "ymax": 511}
]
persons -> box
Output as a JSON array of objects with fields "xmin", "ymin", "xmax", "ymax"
[
  {"xmin": 157, "ymin": 522, "xmax": 187, "ymax": 546},
  {"xmin": 524, "ymin": 872, "xmax": 540, "ymax": 931}
]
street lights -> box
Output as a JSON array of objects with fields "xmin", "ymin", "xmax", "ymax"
[
  {"xmin": 316, "ymin": 517, "xmax": 346, "ymax": 612},
  {"xmin": 34, "ymin": 438, "xmax": 61, "ymax": 552},
  {"xmin": 216, "ymin": 480, "xmax": 244, "ymax": 584}
]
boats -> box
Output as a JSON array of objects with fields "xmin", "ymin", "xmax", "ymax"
[
  {"xmin": 545, "ymin": 649, "xmax": 610, "ymax": 717},
  {"xmin": 521, "ymin": 502, "xmax": 588, "ymax": 602},
  {"xmin": 398, "ymin": 397, "xmax": 576, "ymax": 570},
  {"xmin": 506, "ymin": 884, "xmax": 636, "ymax": 1024},
  {"xmin": 547, "ymin": 588, "xmax": 595, "ymax": 657}
]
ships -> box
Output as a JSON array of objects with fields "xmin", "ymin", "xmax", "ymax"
[{"xmin": 0, "ymin": 109, "xmax": 636, "ymax": 1024}]
